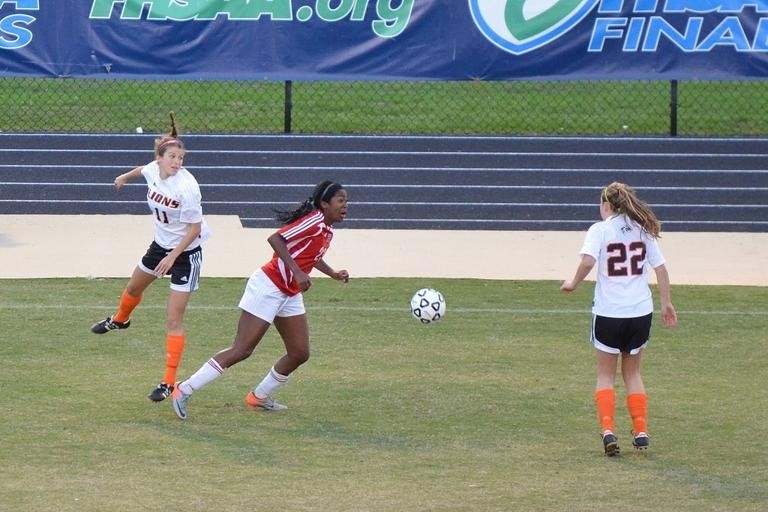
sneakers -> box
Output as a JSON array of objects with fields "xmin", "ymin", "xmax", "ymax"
[
  {"xmin": 92, "ymin": 315, "xmax": 129, "ymax": 334},
  {"xmin": 603, "ymin": 429, "xmax": 620, "ymax": 457},
  {"xmin": 632, "ymin": 431, "xmax": 649, "ymax": 450},
  {"xmin": 173, "ymin": 382, "xmax": 192, "ymax": 420},
  {"xmin": 246, "ymin": 392, "xmax": 286, "ymax": 410},
  {"xmin": 149, "ymin": 383, "xmax": 174, "ymax": 402}
]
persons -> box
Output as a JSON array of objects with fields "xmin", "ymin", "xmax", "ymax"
[
  {"xmin": 560, "ymin": 182, "xmax": 679, "ymax": 454},
  {"xmin": 89, "ymin": 111, "xmax": 212, "ymax": 401},
  {"xmin": 174, "ymin": 180, "xmax": 349, "ymax": 420}
]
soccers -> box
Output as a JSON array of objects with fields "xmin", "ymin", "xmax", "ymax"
[{"xmin": 411, "ymin": 290, "xmax": 446, "ymax": 322}]
